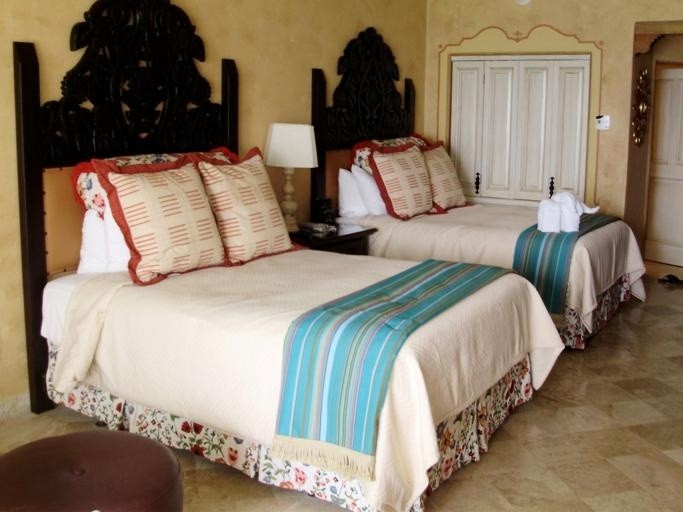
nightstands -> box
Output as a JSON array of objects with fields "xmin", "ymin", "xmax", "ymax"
[{"xmin": 290, "ymin": 219, "xmax": 377, "ymax": 258}]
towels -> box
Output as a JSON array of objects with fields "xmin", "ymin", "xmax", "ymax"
[{"xmin": 534, "ymin": 190, "xmax": 599, "ymax": 235}]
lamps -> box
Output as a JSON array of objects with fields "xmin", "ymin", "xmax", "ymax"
[{"xmin": 262, "ymin": 122, "xmax": 320, "ymax": 232}]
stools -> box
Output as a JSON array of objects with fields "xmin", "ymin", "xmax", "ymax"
[{"xmin": 1, "ymin": 427, "xmax": 183, "ymax": 512}]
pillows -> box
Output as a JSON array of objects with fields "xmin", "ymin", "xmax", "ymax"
[
  {"xmin": 337, "ymin": 133, "xmax": 471, "ymax": 219},
  {"xmin": 71, "ymin": 145, "xmax": 304, "ymax": 288}
]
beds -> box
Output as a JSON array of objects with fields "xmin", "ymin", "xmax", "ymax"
[
  {"xmin": 308, "ymin": 25, "xmax": 647, "ymax": 352},
  {"xmin": 11, "ymin": 0, "xmax": 564, "ymax": 512}
]
